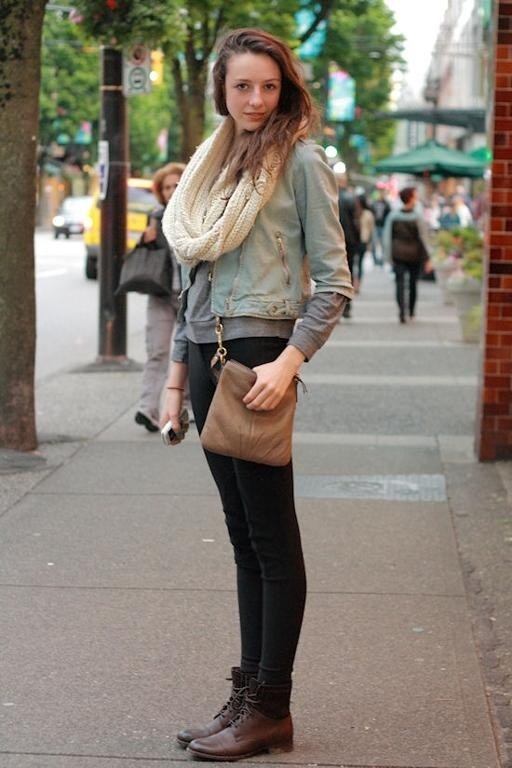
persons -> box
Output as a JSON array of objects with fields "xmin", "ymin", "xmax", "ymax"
[
  {"xmin": 336, "ymin": 172, "xmax": 483, "ymax": 322},
  {"xmin": 159, "ymin": 29, "xmax": 354, "ymax": 762},
  {"xmin": 135, "ymin": 162, "xmax": 196, "ymax": 432}
]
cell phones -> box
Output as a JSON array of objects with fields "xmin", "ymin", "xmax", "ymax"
[{"xmin": 161, "ymin": 407, "xmax": 191, "ymax": 446}]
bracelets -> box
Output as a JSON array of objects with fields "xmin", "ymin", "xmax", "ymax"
[{"xmin": 164, "ymin": 386, "xmax": 184, "ymax": 391}]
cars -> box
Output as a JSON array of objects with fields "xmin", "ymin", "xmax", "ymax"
[{"xmin": 55, "ymin": 196, "xmax": 91, "ymax": 241}]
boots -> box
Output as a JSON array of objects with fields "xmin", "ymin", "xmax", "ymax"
[
  {"xmin": 187, "ymin": 678, "xmax": 296, "ymax": 761},
  {"xmin": 178, "ymin": 666, "xmax": 257, "ymax": 746}
]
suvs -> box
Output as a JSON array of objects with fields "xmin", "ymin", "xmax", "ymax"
[{"xmin": 84, "ymin": 177, "xmax": 164, "ymax": 282}]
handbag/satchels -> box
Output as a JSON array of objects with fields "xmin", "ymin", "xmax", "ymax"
[
  {"xmin": 200, "ymin": 358, "xmax": 298, "ymax": 467},
  {"xmin": 111, "ymin": 246, "xmax": 174, "ymax": 301}
]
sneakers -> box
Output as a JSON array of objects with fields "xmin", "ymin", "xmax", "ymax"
[{"xmin": 135, "ymin": 410, "xmax": 160, "ymax": 432}]
